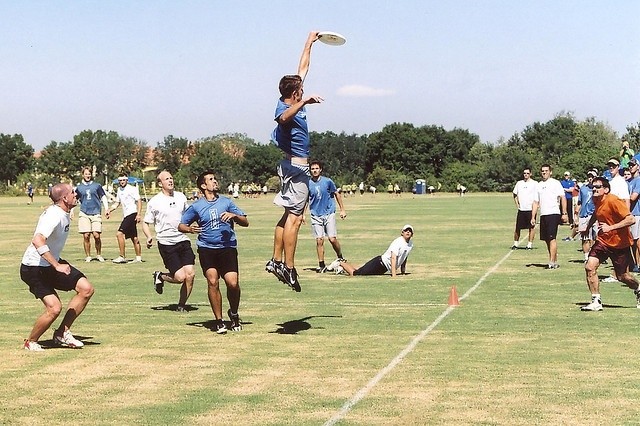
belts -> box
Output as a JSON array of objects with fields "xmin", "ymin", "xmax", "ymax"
[{"xmin": 378, "ymin": 256, "xmax": 386, "ymax": 267}]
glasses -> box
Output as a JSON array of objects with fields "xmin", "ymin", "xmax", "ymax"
[
  {"xmin": 405, "ymin": 229, "xmax": 412, "ymax": 231},
  {"xmin": 592, "ymin": 185, "xmax": 605, "ymax": 188},
  {"xmin": 524, "ymin": 173, "xmax": 529, "ymax": 174}
]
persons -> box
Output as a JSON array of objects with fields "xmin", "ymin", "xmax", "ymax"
[
  {"xmin": 530, "ymin": 164, "xmax": 569, "ymax": 270},
  {"xmin": 142, "ymin": 168, "xmax": 197, "ymax": 314},
  {"xmin": 561, "ymin": 223, "xmax": 579, "ymax": 242},
  {"xmin": 184, "ymin": 180, "xmax": 468, "ymax": 201},
  {"xmin": 177, "ymin": 171, "xmax": 250, "ymax": 336},
  {"xmin": 71, "ymin": 165, "xmax": 110, "ymax": 264},
  {"xmin": 559, "ymin": 171, "xmax": 575, "ymax": 225},
  {"xmin": 327, "ymin": 225, "xmax": 415, "ymax": 278},
  {"xmin": 264, "ymin": 30, "xmax": 325, "ymax": 292},
  {"xmin": 21, "ymin": 181, "xmax": 96, "ymax": 352},
  {"xmin": 512, "ymin": 167, "xmax": 538, "ymax": 251},
  {"xmin": 572, "ymin": 178, "xmax": 581, "ymax": 221},
  {"xmin": 48, "ymin": 182, "xmax": 55, "ymax": 198},
  {"xmin": 577, "ymin": 141, "xmax": 640, "ymax": 311},
  {"xmin": 301, "ymin": 159, "xmax": 347, "ymax": 273},
  {"xmin": 26, "ymin": 182, "xmax": 35, "ymax": 206},
  {"xmin": 106, "ymin": 172, "xmax": 146, "ymax": 266}
]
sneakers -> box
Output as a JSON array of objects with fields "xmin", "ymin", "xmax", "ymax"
[
  {"xmin": 317, "ymin": 265, "xmax": 326, "ymax": 273},
  {"xmin": 113, "ymin": 255, "xmax": 127, "ymax": 263},
  {"xmin": 152, "ymin": 271, "xmax": 164, "ymax": 294},
  {"xmin": 177, "ymin": 304, "xmax": 191, "ymax": 312},
  {"xmin": 53, "ymin": 330, "xmax": 84, "ymax": 348},
  {"xmin": 228, "ymin": 309, "xmax": 242, "ymax": 332},
  {"xmin": 216, "ymin": 319, "xmax": 227, "ymax": 333},
  {"xmin": 276, "ymin": 263, "xmax": 301, "ymax": 292},
  {"xmin": 265, "ymin": 258, "xmax": 288, "ymax": 284},
  {"xmin": 24, "ymin": 340, "xmax": 44, "ymax": 351},
  {"xmin": 512, "ymin": 245, "xmax": 518, "ymax": 250},
  {"xmin": 635, "ymin": 290, "xmax": 640, "ymax": 308},
  {"xmin": 85, "ymin": 257, "xmax": 92, "ymax": 262},
  {"xmin": 128, "ymin": 259, "xmax": 142, "ymax": 263},
  {"xmin": 97, "ymin": 255, "xmax": 105, "ymax": 261},
  {"xmin": 554, "ymin": 264, "xmax": 560, "ymax": 269},
  {"xmin": 327, "ymin": 258, "xmax": 343, "ymax": 270},
  {"xmin": 602, "ymin": 276, "xmax": 618, "ymax": 282},
  {"xmin": 545, "ymin": 264, "xmax": 554, "ymax": 269},
  {"xmin": 562, "ymin": 237, "xmax": 574, "ymax": 241},
  {"xmin": 526, "ymin": 246, "xmax": 532, "ymax": 250},
  {"xmin": 581, "ymin": 296, "xmax": 603, "ymax": 311}
]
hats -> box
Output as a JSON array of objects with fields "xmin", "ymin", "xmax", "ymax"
[
  {"xmin": 117, "ymin": 177, "xmax": 128, "ymax": 180},
  {"xmin": 564, "ymin": 171, "xmax": 569, "ymax": 175},
  {"xmin": 608, "ymin": 159, "xmax": 619, "ymax": 165},
  {"xmin": 627, "ymin": 159, "xmax": 639, "ymax": 165},
  {"xmin": 402, "ymin": 225, "xmax": 414, "ymax": 233},
  {"xmin": 587, "ymin": 171, "xmax": 597, "ymax": 176}
]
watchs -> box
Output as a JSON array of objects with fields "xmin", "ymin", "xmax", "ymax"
[{"xmin": 562, "ymin": 213, "xmax": 568, "ymax": 215}]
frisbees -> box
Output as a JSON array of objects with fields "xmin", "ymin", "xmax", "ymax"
[{"xmin": 318, "ymin": 31, "xmax": 346, "ymax": 47}]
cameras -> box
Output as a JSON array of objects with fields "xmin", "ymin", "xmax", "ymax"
[{"xmin": 622, "ymin": 146, "xmax": 627, "ymax": 154}]
